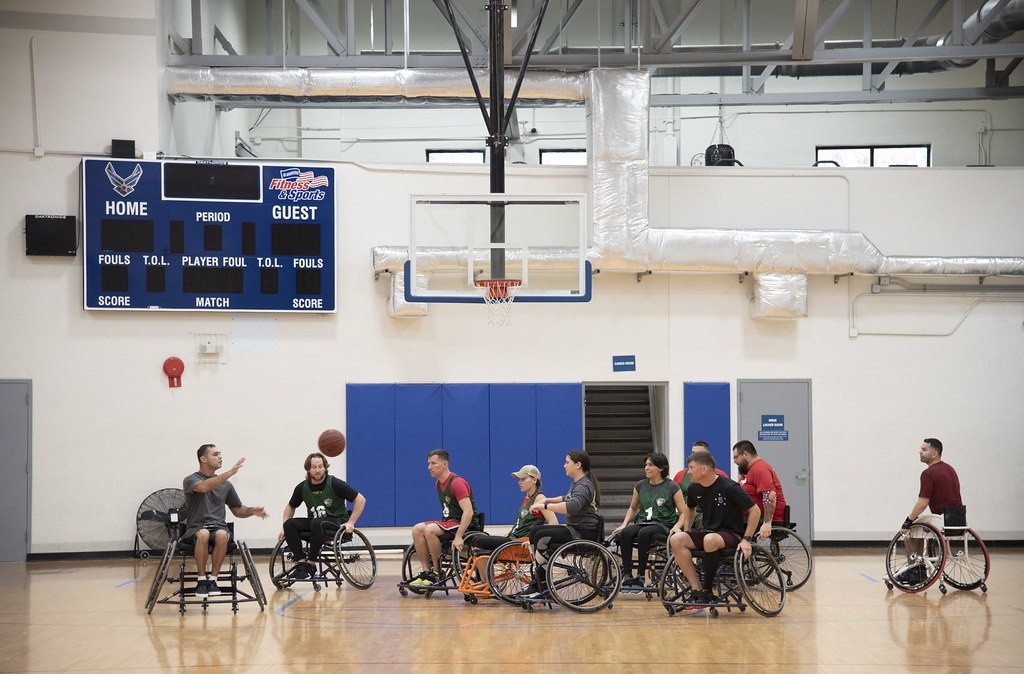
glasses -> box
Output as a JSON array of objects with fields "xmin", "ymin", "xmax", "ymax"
[{"xmin": 733, "ymin": 450, "xmax": 745, "ymax": 460}]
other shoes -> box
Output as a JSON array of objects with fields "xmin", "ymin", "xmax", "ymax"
[{"xmin": 472, "ymin": 579, "xmax": 499, "ymax": 593}]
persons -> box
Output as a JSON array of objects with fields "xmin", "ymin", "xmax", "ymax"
[
  {"xmin": 277, "ymin": 453, "xmax": 366, "ymax": 579},
  {"xmin": 182, "ymin": 444, "xmax": 268, "ymax": 597},
  {"xmin": 673, "ymin": 441, "xmax": 729, "ymax": 530},
  {"xmin": 472, "ymin": 464, "xmax": 559, "ymax": 582},
  {"xmin": 902, "ymin": 438, "xmax": 962, "ymax": 579},
  {"xmin": 520, "ymin": 451, "xmax": 601, "ymax": 597},
  {"xmin": 410, "ymin": 449, "xmax": 479, "ymax": 587},
  {"xmin": 670, "ymin": 451, "xmax": 761, "ymax": 610},
  {"xmin": 612, "ymin": 453, "xmax": 686, "ymax": 595},
  {"xmin": 733, "ymin": 440, "xmax": 787, "ymax": 539}
]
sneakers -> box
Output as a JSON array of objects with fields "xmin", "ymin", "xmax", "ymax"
[
  {"xmin": 207, "ymin": 580, "xmax": 221, "ymax": 596},
  {"xmin": 694, "ymin": 590, "xmax": 710, "ymax": 609},
  {"xmin": 195, "ymin": 580, "xmax": 208, "ymax": 597},
  {"xmin": 685, "ymin": 589, "xmax": 704, "ymax": 610},
  {"xmin": 621, "ymin": 573, "xmax": 634, "ymax": 595},
  {"xmin": 296, "ymin": 563, "xmax": 319, "ymax": 582},
  {"xmin": 409, "ymin": 569, "xmax": 432, "ymax": 585},
  {"xmin": 422, "ymin": 570, "xmax": 445, "ymax": 585},
  {"xmin": 630, "ymin": 576, "xmax": 647, "ymax": 595},
  {"xmin": 519, "ymin": 581, "xmax": 551, "ymax": 598},
  {"xmin": 287, "ymin": 561, "xmax": 305, "ymax": 580}
]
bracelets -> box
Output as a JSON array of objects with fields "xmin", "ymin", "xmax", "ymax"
[{"xmin": 545, "ymin": 503, "xmax": 547, "ymax": 509}]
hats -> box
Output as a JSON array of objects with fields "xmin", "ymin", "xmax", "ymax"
[{"xmin": 511, "ymin": 464, "xmax": 541, "ymax": 480}]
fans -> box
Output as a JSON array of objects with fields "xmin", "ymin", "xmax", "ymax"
[{"xmin": 135, "ymin": 488, "xmax": 189, "ymax": 552}]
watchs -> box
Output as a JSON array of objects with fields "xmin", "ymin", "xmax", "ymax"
[{"xmin": 743, "ymin": 536, "xmax": 752, "ymax": 541}]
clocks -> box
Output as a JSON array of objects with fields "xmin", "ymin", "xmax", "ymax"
[{"xmin": 690, "ymin": 153, "xmax": 706, "ymax": 167}]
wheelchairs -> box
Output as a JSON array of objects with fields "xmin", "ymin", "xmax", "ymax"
[
  {"xmin": 884, "ymin": 505, "xmax": 991, "ymax": 595},
  {"xmin": 485, "ymin": 514, "xmax": 623, "ymax": 614},
  {"xmin": 144, "ymin": 521, "xmax": 267, "ymax": 615},
  {"xmin": 397, "ymin": 512, "xmax": 491, "ymax": 600},
  {"xmin": 590, "ymin": 504, "xmax": 813, "ymax": 619},
  {"xmin": 268, "ymin": 510, "xmax": 378, "ymax": 590},
  {"xmin": 457, "ymin": 524, "xmax": 549, "ymax": 605}
]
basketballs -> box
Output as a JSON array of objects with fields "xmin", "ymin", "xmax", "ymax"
[{"xmin": 318, "ymin": 429, "xmax": 346, "ymax": 457}]
